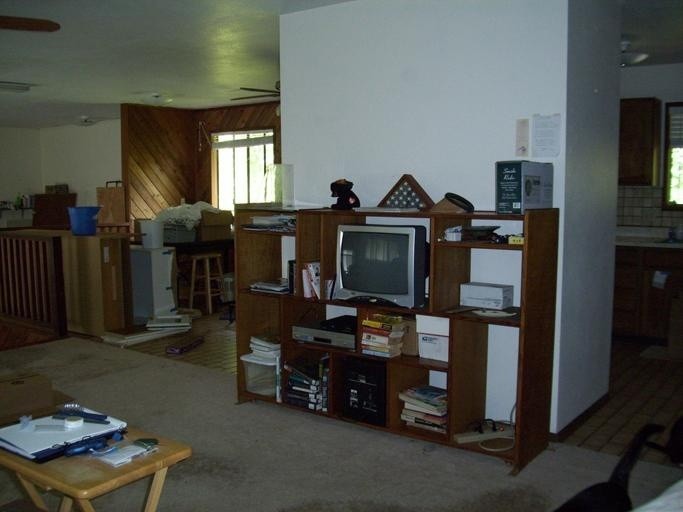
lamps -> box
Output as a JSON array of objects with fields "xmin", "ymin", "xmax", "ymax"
[{"xmin": 617, "ymin": 33, "xmax": 650, "ymax": 69}]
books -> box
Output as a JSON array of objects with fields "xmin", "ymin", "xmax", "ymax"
[
  {"xmin": 0, "ymin": 406, "xmax": 127, "ymax": 463},
  {"xmin": 282, "ymin": 356, "xmax": 328, "ymax": 413},
  {"xmin": 248, "ymin": 335, "xmax": 280, "ymax": 359},
  {"xmin": 360, "ymin": 315, "xmax": 404, "ymax": 361},
  {"xmin": 399, "ymin": 382, "xmax": 447, "ymax": 436},
  {"xmin": 249, "ymin": 259, "xmax": 321, "ymax": 300}
]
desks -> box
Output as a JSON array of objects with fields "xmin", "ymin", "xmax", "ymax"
[{"xmin": 0, "ymin": 425, "xmax": 195, "ymax": 512}]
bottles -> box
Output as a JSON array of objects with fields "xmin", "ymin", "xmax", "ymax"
[{"xmin": 14, "ymin": 192, "xmax": 30, "ymax": 208}]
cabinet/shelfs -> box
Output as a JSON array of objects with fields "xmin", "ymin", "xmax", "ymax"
[
  {"xmin": 613, "ymin": 245, "xmax": 683, "ymax": 337},
  {"xmin": 235, "ymin": 203, "xmax": 560, "ymax": 478},
  {"xmin": 0, "ymin": 230, "xmax": 148, "ymax": 338},
  {"xmin": 618, "ymin": 99, "xmax": 661, "ymax": 185}
]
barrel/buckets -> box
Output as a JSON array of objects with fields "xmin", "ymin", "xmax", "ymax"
[{"xmin": 67, "ymin": 205, "xmax": 104, "ymax": 235}]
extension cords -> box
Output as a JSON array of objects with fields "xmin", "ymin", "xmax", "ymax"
[{"xmin": 453, "ymin": 426, "xmax": 515, "ymax": 444}]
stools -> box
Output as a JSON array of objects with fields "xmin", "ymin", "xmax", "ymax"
[{"xmin": 190, "ymin": 254, "xmax": 227, "ymax": 314}]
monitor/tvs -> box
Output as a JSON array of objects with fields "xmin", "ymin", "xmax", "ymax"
[{"xmin": 332, "ymin": 224, "xmax": 430, "ymax": 309}]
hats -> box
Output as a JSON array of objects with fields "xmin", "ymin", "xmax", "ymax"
[{"xmin": 431, "ymin": 193, "xmax": 474, "ymax": 214}]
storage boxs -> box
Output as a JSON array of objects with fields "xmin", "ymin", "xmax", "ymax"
[
  {"xmin": 199, "ymin": 208, "xmax": 234, "ymax": 242},
  {"xmin": 0, "ymin": 371, "xmax": 54, "ymax": 417},
  {"xmin": 239, "ymin": 352, "xmax": 279, "ymax": 397}
]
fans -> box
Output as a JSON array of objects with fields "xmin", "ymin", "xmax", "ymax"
[{"xmin": 229, "ymin": 79, "xmax": 280, "ymax": 104}]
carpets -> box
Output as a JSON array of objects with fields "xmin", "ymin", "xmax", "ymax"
[{"xmin": 0, "ymin": 335, "xmax": 683, "ymax": 512}]
